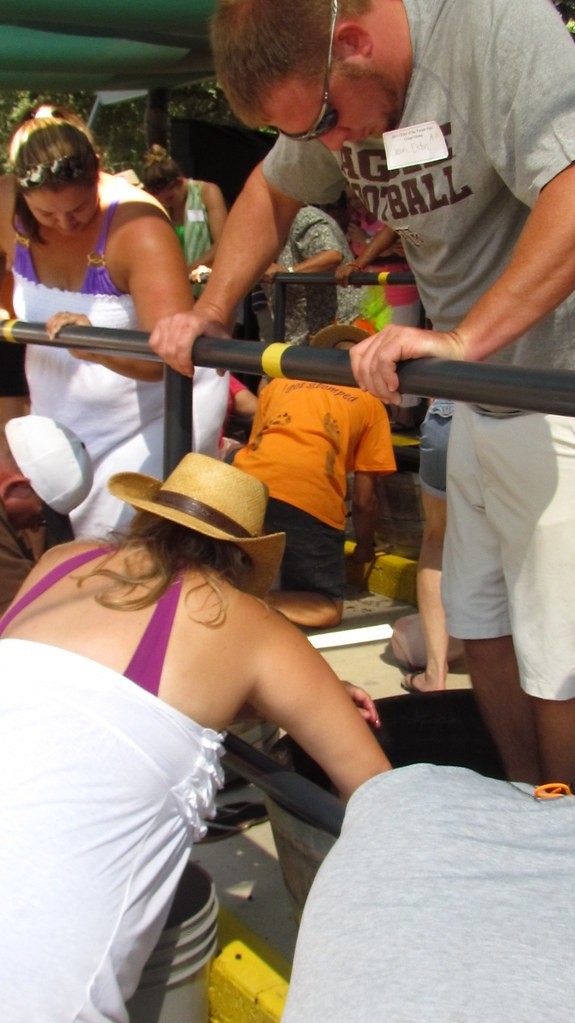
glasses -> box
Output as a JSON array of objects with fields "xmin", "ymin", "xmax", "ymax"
[
  {"xmin": 260, "ymin": 0, "xmax": 338, "ymax": 143},
  {"xmin": 15, "ymin": 155, "xmax": 93, "ymax": 189}
]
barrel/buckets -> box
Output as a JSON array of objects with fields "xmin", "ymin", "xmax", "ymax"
[
  {"xmin": 125, "ymin": 858, "xmax": 219, "ymax": 1023},
  {"xmin": 266, "ymin": 687, "xmax": 510, "ymax": 929}
]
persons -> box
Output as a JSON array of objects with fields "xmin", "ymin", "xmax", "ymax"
[{"xmin": 0, "ymin": 0, "xmax": 575, "ymax": 1023}]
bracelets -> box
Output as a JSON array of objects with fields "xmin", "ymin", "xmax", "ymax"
[
  {"xmin": 346, "ymin": 263, "xmax": 362, "ymax": 270},
  {"xmin": 287, "ymin": 264, "xmax": 294, "ymax": 272}
]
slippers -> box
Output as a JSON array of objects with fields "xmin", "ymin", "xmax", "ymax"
[{"xmin": 400, "ymin": 671, "xmax": 426, "ymax": 694}]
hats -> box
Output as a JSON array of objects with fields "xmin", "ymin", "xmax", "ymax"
[
  {"xmin": 106, "ymin": 449, "xmax": 286, "ymax": 601},
  {"xmin": 5, "ymin": 414, "xmax": 94, "ymax": 542},
  {"xmin": 311, "ymin": 323, "xmax": 372, "ymax": 352}
]
